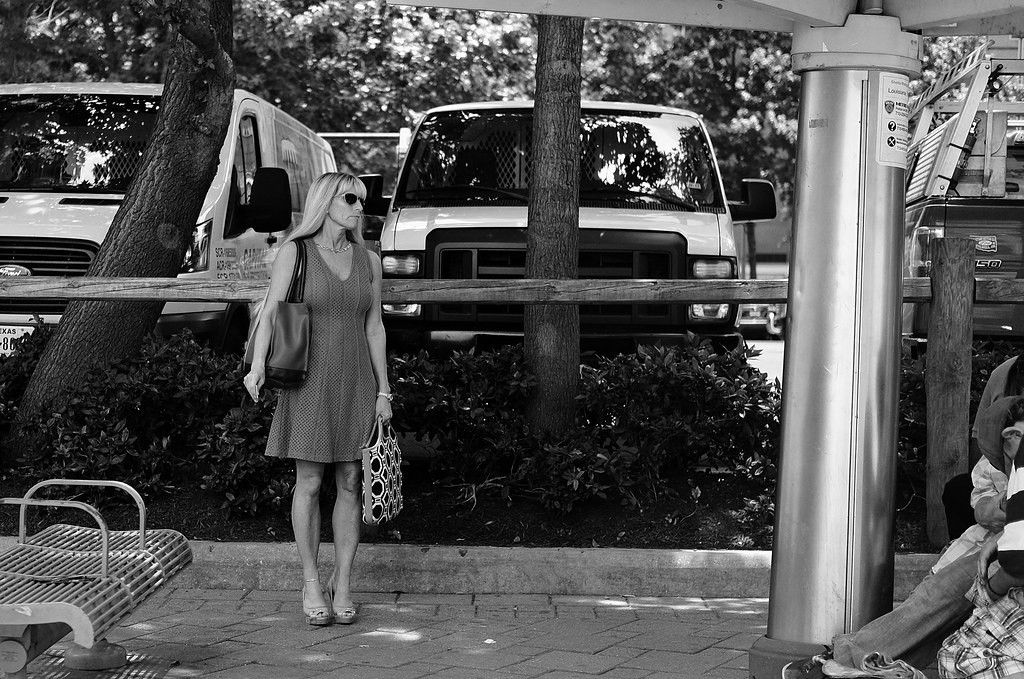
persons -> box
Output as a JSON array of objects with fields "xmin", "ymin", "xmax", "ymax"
[
  {"xmin": 781, "ymin": 354, "xmax": 1024, "ymax": 679},
  {"xmin": 243, "ymin": 173, "xmax": 392, "ymax": 625}
]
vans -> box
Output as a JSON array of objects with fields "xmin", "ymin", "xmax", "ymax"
[
  {"xmin": 0, "ymin": 81, "xmax": 337, "ymax": 360},
  {"xmin": 356, "ymin": 99, "xmax": 779, "ymax": 361}
]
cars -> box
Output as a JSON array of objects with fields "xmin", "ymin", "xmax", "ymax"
[{"xmin": 899, "ymin": 141, "xmax": 1024, "ymax": 341}]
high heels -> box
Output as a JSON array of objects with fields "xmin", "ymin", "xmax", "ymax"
[
  {"xmin": 302, "ymin": 579, "xmax": 329, "ymax": 624},
  {"xmin": 326, "ymin": 578, "xmax": 356, "ymax": 624}
]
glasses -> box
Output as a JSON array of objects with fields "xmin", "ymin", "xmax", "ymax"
[{"xmin": 331, "ymin": 192, "xmax": 365, "ymax": 207}]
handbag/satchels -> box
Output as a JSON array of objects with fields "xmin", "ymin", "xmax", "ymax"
[
  {"xmin": 243, "ymin": 238, "xmax": 312, "ymax": 389},
  {"xmin": 360, "ymin": 414, "xmax": 403, "ymax": 525}
]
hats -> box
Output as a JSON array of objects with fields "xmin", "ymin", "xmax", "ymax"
[{"xmin": 976, "ymin": 396, "xmax": 1024, "ymax": 474}]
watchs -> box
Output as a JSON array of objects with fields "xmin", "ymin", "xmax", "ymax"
[{"xmin": 377, "ymin": 392, "xmax": 393, "ymax": 401}]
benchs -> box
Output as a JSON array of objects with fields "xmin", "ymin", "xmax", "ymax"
[{"xmin": 0, "ymin": 479, "xmax": 193, "ymax": 679}]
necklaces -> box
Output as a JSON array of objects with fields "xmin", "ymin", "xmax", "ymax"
[{"xmin": 316, "ymin": 244, "xmax": 351, "ymax": 253}]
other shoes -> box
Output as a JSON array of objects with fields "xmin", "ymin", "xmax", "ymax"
[{"xmin": 782, "ymin": 643, "xmax": 835, "ymax": 679}]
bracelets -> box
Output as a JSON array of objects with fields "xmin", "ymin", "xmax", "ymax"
[{"xmin": 988, "ymin": 575, "xmax": 1007, "ymax": 596}]
